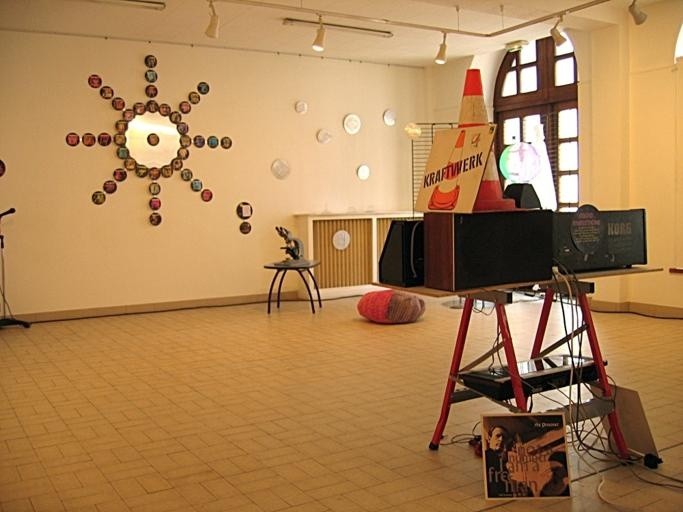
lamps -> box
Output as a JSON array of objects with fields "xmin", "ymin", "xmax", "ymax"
[
  {"xmin": 205, "ymin": 14, "xmax": 221, "ymax": 38},
  {"xmin": 312, "ymin": 28, "xmax": 326, "ymax": 52},
  {"xmin": 435, "ymin": 43, "xmax": 447, "ymax": 64},
  {"xmin": 628, "ymin": 4, "xmax": 647, "ymax": 24},
  {"xmin": 550, "ymin": 28, "xmax": 566, "ymax": 46}
]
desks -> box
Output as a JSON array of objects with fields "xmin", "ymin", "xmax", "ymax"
[
  {"xmin": 264, "ymin": 259, "xmax": 322, "ymax": 314},
  {"xmin": 368, "ymin": 264, "xmax": 663, "ymax": 465}
]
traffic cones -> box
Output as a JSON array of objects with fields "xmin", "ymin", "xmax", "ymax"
[
  {"xmin": 425, "ymin": 130, "xmax": 468, "ymax": 215},
  {"xmin": 445, "ymin": 67, "xmax": 517, "ymax": 211}
]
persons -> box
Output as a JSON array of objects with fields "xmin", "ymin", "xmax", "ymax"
[{"xmin": 485, "ymin": 425, "xmax": 513, "ymax": 497}]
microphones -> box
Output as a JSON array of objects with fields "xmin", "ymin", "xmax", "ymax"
[{"xmin": 0, "ymin": 208, "xmax": 15, "ymax": 217}]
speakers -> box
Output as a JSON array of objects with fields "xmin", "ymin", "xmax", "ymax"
[
  {"xmin": 424, "ymin": 209, "xmax": 552, "ymax": 291},
  {"xmin": 379, "ymin": 221, "xmax": 424, "ymax": 287}
]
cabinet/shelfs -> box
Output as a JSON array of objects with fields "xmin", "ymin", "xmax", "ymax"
[{"xmin": 293, "ymin": 211, "xmax": 424, "ymax": 302}]
